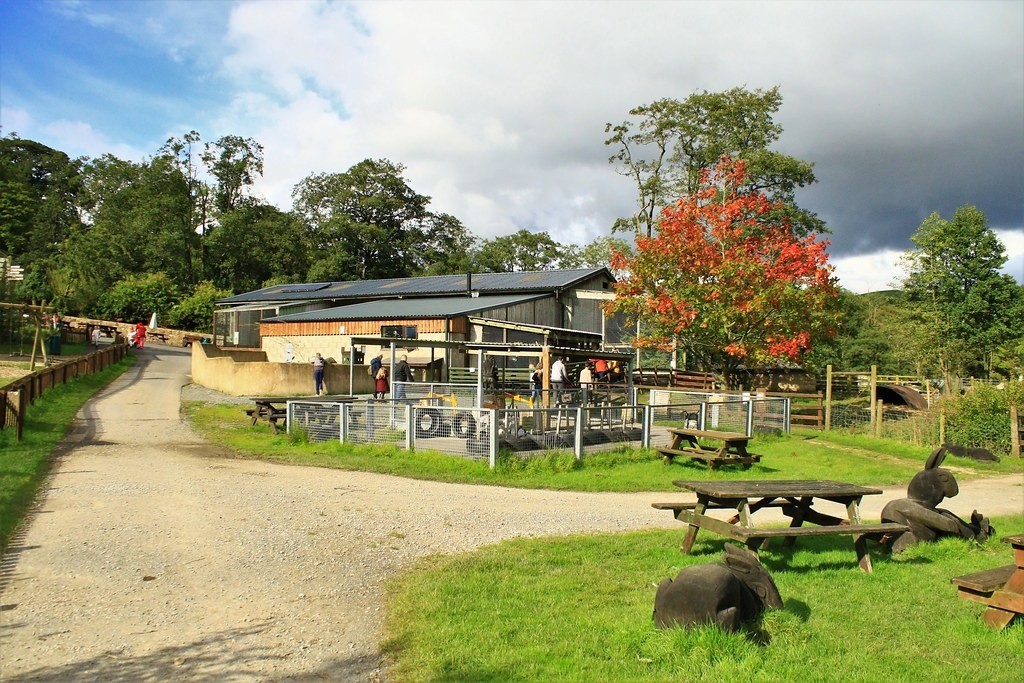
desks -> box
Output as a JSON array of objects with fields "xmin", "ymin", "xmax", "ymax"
[
  {"xmin": 651, "ymin": 480, "xmax": 910, "ymax": 573},
  {"xmin": 657, "ymin": 427, "xmax": 762, "ymax": 470}
]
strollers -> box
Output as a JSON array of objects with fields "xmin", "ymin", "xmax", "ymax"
[{"xmin": 561, "ymin": 376, "xmax": 580, "ymax": 404}]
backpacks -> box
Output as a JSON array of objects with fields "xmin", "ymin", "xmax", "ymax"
[{"xmin": 367, "ymin": 365, "xmax": 373, "ymax": 375}]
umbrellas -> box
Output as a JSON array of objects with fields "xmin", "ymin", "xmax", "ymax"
[{"xmin": 149, "ymin": 312, "xmax": 158, "ymax": 332}]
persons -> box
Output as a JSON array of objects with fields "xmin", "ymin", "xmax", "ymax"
[
  {"xmin": 310, "ymin": 353, "xmax": 328, "ymax": 396},
  {"xmin": 367, "ymin": 353, "xmax": 390, "ymax": 403},
  {"xmin": 551, "ymin": 356, "xmax": 570, "ymax": 408},
  {"xmin": 126, "ymin": 321, "xmax": 147, "ymax": 351},
  {"xmin": 579, "ymin": 362, "xmax": 596, "ymax": 406},
  {"xmin": 392, "ymin": 354, "xmax": 415, "ymax": 407},
  {"xmin": 91, "ymin": 324, "xmax": 101, "ymax": 351},
  {"xmin": 529, "ymin": 362, "xmax": 543, "ymax": 409}
]
